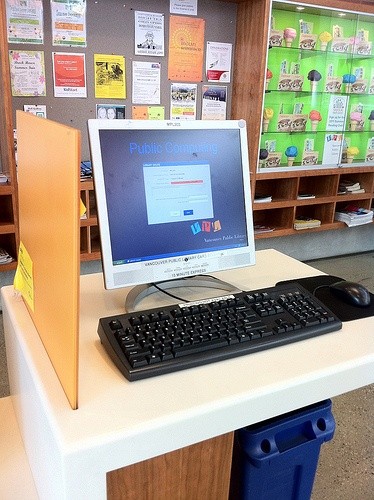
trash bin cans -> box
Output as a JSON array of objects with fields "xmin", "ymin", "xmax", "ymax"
[{"xmin": 229, "ymin": 399, "xmax": 336, "ymax": 500}]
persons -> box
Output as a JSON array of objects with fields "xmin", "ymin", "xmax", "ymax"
[
  {"xmin": 106, "ymin": 108, "xmax": 116, "ymax": 119},
  {"xmin": 98, "ymin": 108, "xmax": 106, "ymax": 119}
]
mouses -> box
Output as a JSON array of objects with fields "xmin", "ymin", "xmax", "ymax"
[{"xmin": 330, "ymin": 280, "xmax": 371, "ymax": 307}]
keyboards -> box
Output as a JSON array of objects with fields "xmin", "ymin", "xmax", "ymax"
[{"xmin": 97, "ymin": 283, "xmax": 343, "ymax": 381}]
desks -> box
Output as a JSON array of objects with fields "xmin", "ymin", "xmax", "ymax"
[{"xmin": 1, "ymin": 248, "xmax": 374, "ymax": 500}]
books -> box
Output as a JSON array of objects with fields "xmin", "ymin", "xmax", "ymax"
[
  {"xmin": 254, "ymin": 195, "xmax": 272, "ymax": 203},
  {"xmin": 335, "ymin": 207, "xmax": 373, "ymax": 227},
  {"xmin": 294, "ymin": 218, "xmax": 321, "ymax": 230},
  {"xmin": 298, "ymin": 193, "xmax": 315, "ymax": 200},
  {"xmin": 0, "ymin": 249, "xmax": 14, "ymax": 264},
  {"xmin": 338, "ymin": 182, "xmax": 365, "ymax": 195}
]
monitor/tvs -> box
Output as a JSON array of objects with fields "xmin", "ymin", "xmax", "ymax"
[{"xmin": 86, "ymin": 118, "xmax": 257, "ymax": 313}]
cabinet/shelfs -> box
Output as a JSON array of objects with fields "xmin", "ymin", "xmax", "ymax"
[{"xmin": 0, "ymin": 0, "xmax": 374, "ymax": 271}]
